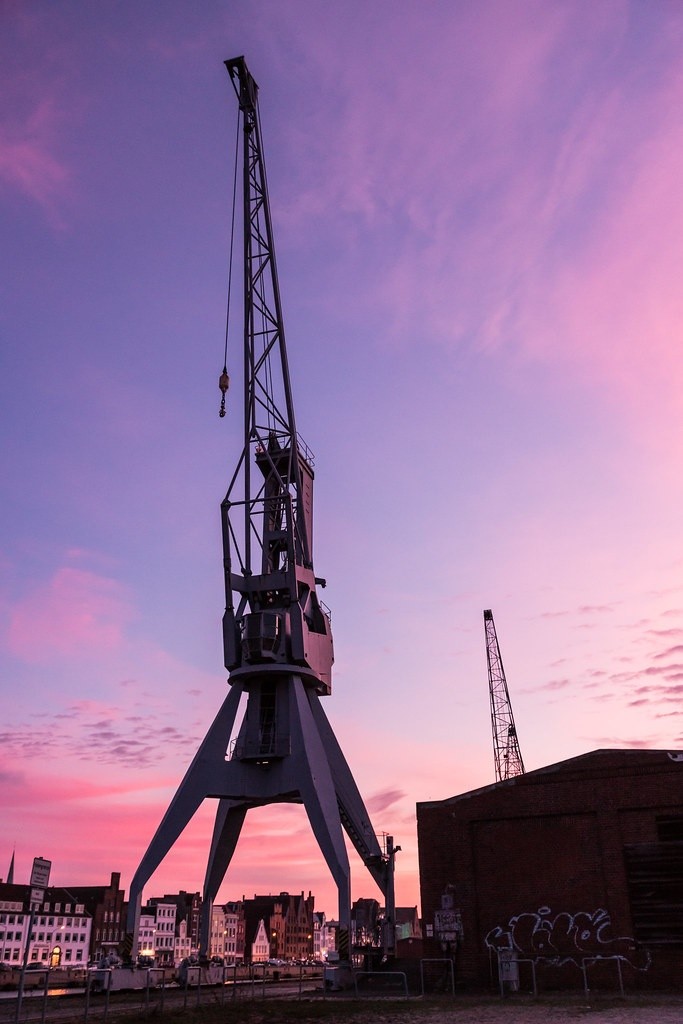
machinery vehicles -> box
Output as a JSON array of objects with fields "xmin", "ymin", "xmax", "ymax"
[
  {"xmin": 483, "ymin": 609, "xmax": 526, "ymax": 788},
  {"xmin": 219, "ymin": 54, "xmax": 336, "ymax": 692}
]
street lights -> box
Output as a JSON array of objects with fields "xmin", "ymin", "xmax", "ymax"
[{"xmin": 48, "ymin": 925, "xmax": 65, "ymax": 966}]
